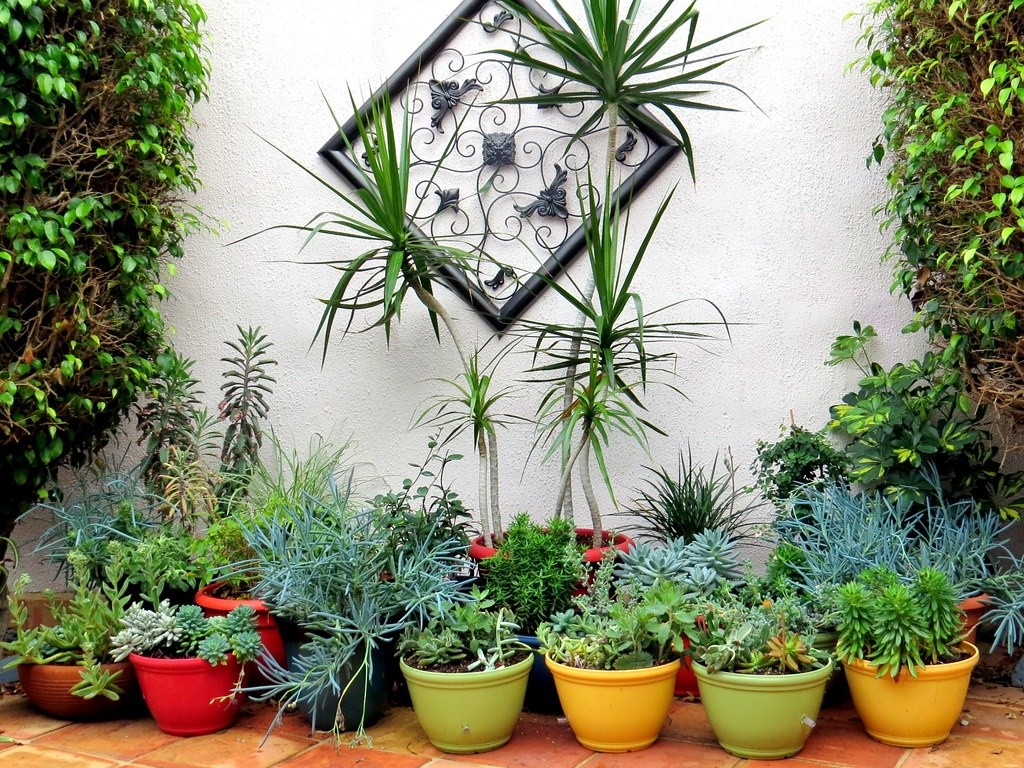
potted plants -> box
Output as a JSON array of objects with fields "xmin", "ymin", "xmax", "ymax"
[
  {"xmin": 224, "ymin": 0, "xmax": 769, "ymax": 597},
  {"xmin": 691, "ymin": 603, "xmax": 829, "ymax": 761},
  {"xmin": 223, "ymin": 496, "xmax": 473, "ymax": 751},
  {"xmin": 393, "ymin": 584, "xmax": 533, "ymax": 755},
  {"xmin": 616, "ymin": 529, "xmax": 748, "ymax": 696},
  {"xmin": 0, "ymin": 549, "xmax": 135, "ymax": 720},
  {"xmin": 831, "ymin": 568, "xmax": 979, "ymax": 752},
  {"xmin": 534, "ymin": 535, "xmax": 699, "ymax": 753},
  {"xmin": 109, "ymin": 600, "xmax": 264, "ymax": 738},
  {"xmin": 906, "ymin": 504, "xmax": 1020, "ymax": 646},
  {"xmin": 195, "ymin": 516, "xmax": 294, "ymax": 679}
]
